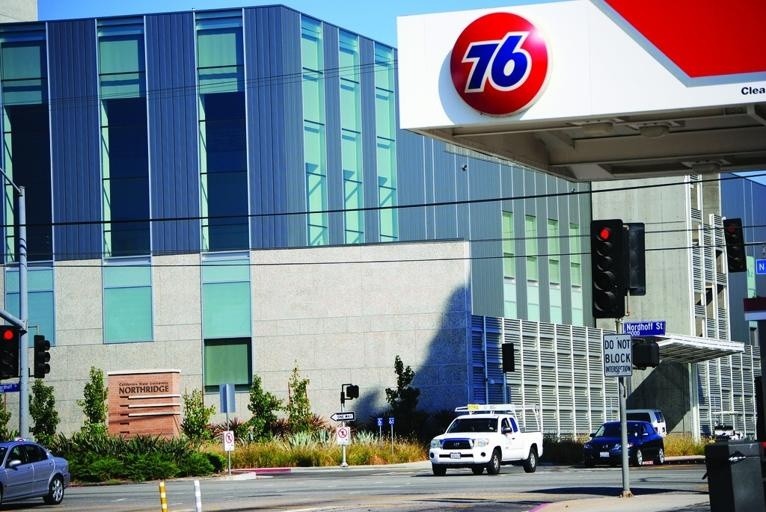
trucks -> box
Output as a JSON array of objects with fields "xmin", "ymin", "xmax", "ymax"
[{"xmin": 428, "ymin": 403, "xmax": 546, "ymax": 478}]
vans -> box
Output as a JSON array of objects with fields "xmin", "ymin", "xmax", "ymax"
[{"xmin": 625, "ymin": 408, "xmax": 669, "ymax": 440}]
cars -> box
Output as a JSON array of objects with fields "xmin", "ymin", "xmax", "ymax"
[
  {"xmin": 583, "ymin": 419, "xmax": 667, "ymax": 469},
  {"xmin": 0, "ymin": 440, "xmax": 69, "ymax": 512}
]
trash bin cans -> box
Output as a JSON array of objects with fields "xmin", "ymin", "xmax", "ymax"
[{"xmin": 704, "ymin": 442, "xmax": 766, "ymax": 512}]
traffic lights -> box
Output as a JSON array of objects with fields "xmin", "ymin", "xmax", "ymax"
[
  {"xmin": 0, "ymin": 325, "xmax": 21, "ymax": 382},
  {"xmin": 347, "ymin": 385, "xmax": 361, "ymax": 400},
  {"xmin": 722, "ymin": 216, "xmax": 748, "ymax": 273},
  {"xmin": 590, "ymin": 219, "xmax": 630, "ymax": 321},
  {"xmin": 34, "ymin": 335, "xmax": 53, "ymax": 380}
]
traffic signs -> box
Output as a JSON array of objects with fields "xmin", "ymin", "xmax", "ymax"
[
  {"xmin": 756, "ymin": 260, "xmax": 766, "ymax": 275},
  {"xmin": 329, "ymin": 412, "xmax": 355, "ymax": 421},
  {"xmin": 623, "ymin": 320, "xmax": 668, "ymax": 338}
]
